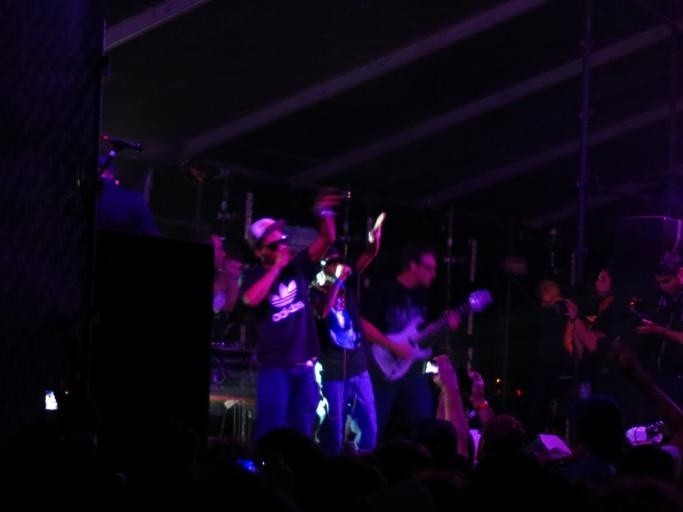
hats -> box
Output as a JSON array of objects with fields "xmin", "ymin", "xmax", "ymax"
[{"xmin": 246, "ymin": 219, "xmax": 284, "ymax": 248}]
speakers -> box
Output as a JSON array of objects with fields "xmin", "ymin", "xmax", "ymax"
[{"xmin": 79, "ymin": 233, "xmax": 212, "ymax": 463}]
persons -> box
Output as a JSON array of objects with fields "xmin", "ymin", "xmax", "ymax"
[
  {"xmin": 97, "ymin": 185, "xmax": 160, "ymax": 233},
  {"xmin": 147, "ymin": 158, "xmax": 202, "ymax": 219},
  {"xmin": 205, "ymin": 187, "xmax": 682, "ymax": 510}
]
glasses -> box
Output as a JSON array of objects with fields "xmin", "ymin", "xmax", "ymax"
[{"xmin": 259, "ymin": 235, "xmax": 287, "ymax": 251}]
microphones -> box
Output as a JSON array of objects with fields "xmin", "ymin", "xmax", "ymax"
[
  {"xmin": 368, "ymin": 211, "xmax": 386, "ymax": 244},
  {"xmin": 101, "ymin": 133, "xmax": 146, "ymax": 153},
  {"xmin": 318, "ymin": 185, "xmax": 352, "ymax": 200}
]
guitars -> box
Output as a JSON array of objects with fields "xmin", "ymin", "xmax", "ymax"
[{"xmin": 372, "ymin": 290, "xmax": 493, "ymax": 382}]
